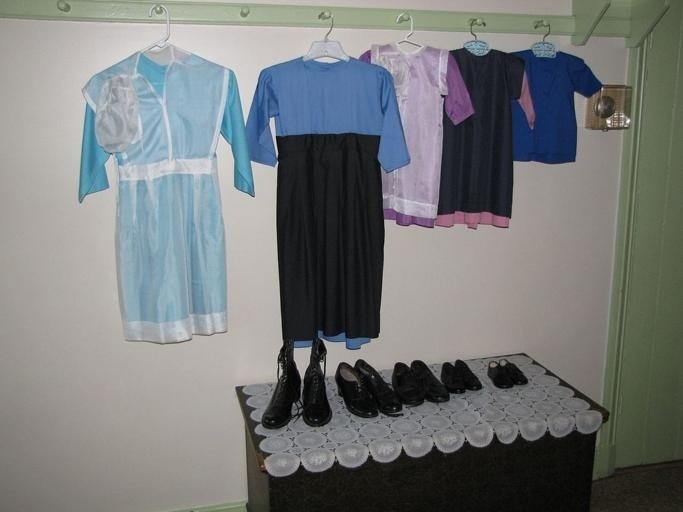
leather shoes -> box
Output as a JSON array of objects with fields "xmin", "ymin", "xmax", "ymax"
[
  {"xmin": 455, "ymin": 359, "xmax": 482, "ymax": 391},
  {"xmin": 499, "ymin": 359, "xmax": 528, "ymax": 386},
  {"xmin": 411, "ymin": 360, "xmax": 450, "ymax": 403},
  {"xmin": 392, "ymin": 362, "xmax": 425, "ymax": 406},
  {"xmin": 441, "ymin": 362, "xmax": 465, "ymax": 394},
  {"xmin": 354, "ymin": 359, "xmax": 402, "ymax": 413},
  {"xmin": 487, "ymin": 361, "xmax": 513, "ymax": 388},
  {"xmin": 335, "ymin": 362, "xmax": 378, "ymax": 418}
]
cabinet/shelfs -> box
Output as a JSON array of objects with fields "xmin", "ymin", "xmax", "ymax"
[{"xmin": 234, "ymin": 351, "xmax": 611, "ymax": 512}]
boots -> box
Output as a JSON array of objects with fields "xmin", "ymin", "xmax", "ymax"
[
  {"xmin": 262, "ymin": 340, "xmax": 303, "ymax": 429},
  {"xmin": 302, "ymin": 337, "xmax": 332, "ymax": 427}
]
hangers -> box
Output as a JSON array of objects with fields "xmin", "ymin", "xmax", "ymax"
[
  {"xmin": 464, "ymin": 18, "xmax": 490, "ymax": 49},
  {"xmin": 394, "ymin": 12, "xmax": 421, "ymax": 50},
  {"xmin": 139, "ymin": 5, "xmax": 189, "ymax": 56},
  {"xmin": 300, "ymin": 9, "xmax": 350, "ymax": 62},
  {"xmin": 530, "ymin": 21, "xmax": 557, "ymax": 52}
]
